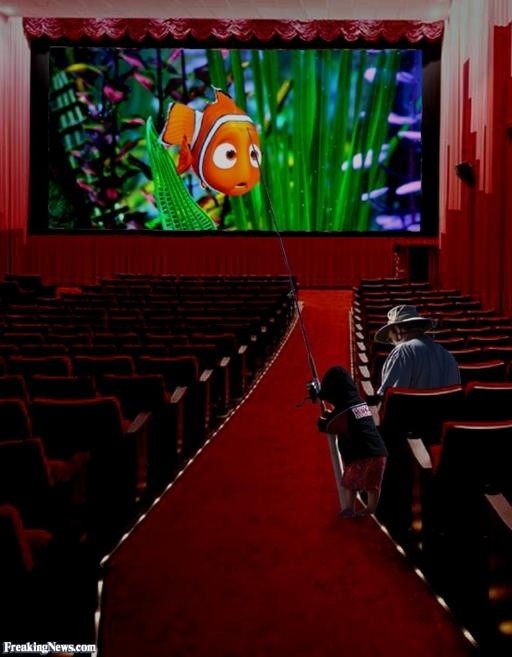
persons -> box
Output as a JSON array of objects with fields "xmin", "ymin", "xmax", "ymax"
[
  {"xmin": 375, "ymin": 304, "xmax": 462, "ymax": 525},
  {"xmin": 307, "ymin": 366, "xmax": 388, "ymax": 519}
]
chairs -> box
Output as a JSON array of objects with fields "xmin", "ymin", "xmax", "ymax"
[
  {"xmin": 350, "ymin": 278, "xmax": 512, "ymax": 657},
  {"xmin": 0, "ymin": 274, "xmax": 301, "ymax": 657}
]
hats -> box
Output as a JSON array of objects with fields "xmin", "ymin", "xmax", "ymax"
[{"xmin": 374, "ymin": 304, "xmax": 433, "ymax": 345}]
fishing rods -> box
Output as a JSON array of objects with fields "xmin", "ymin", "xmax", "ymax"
[{"xmin": 246, "ymin": 127, "xmax": 347, "ymax": 513}]
